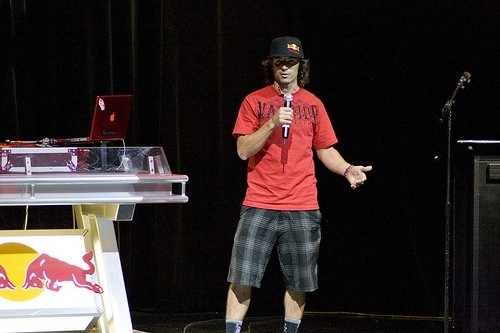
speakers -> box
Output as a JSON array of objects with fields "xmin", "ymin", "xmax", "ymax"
[{"xmin": 452, "ymin": 149, "xmax": 500, "ymax": 333}]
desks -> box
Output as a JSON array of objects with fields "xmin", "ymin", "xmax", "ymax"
[{"xmin": 0, "ymin": 175, "xmax": 189, "ymax": 333}]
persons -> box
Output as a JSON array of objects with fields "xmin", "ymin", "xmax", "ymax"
[{"xmin": 226, "ymin": 36, "xmax": 372, "ymax": 333}]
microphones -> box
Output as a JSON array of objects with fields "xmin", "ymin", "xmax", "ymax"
[
  {"xmin": 442, "ymin": 71, "xmax": 472, "ymax": 115},
  {"xmin": 282, "ymin": 94, "xmax": 293, "ymax": 139}
]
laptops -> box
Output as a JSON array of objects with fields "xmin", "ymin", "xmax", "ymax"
[{"xmin": 55, "ymin": 94, "xmax": 131, "ymax": 144}]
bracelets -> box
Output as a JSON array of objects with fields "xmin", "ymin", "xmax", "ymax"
[{"xmin": 344, "ymin": 165, "xmax": 353, "ymax": 177}]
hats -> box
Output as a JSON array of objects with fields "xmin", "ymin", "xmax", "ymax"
[{"xmin": 270, "ymin": 36, "xmax": 305, "ymax": 58}]
